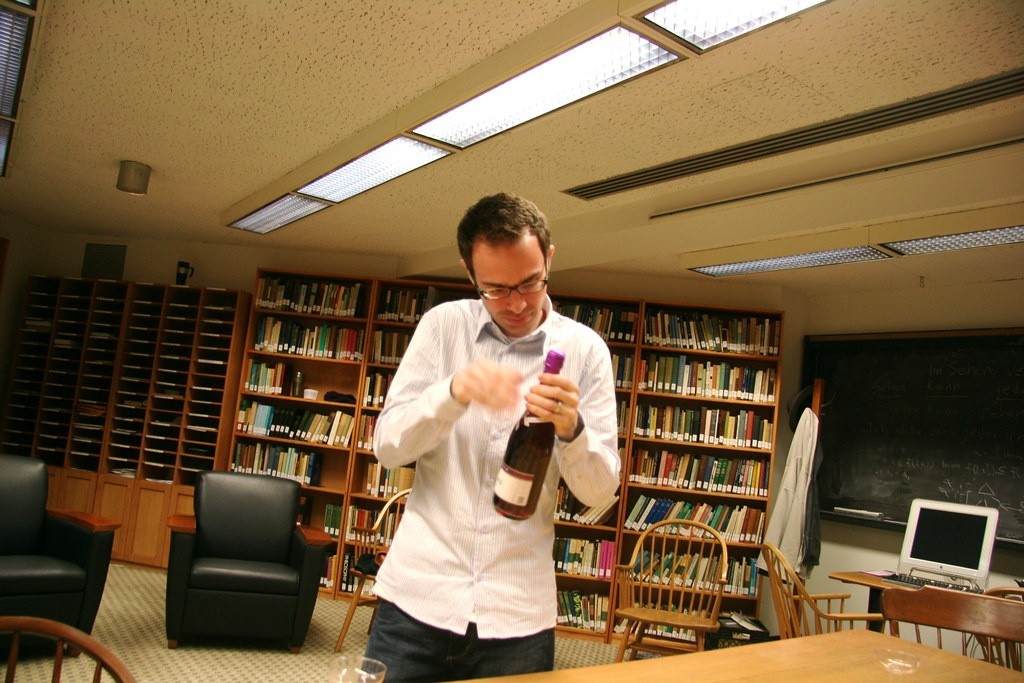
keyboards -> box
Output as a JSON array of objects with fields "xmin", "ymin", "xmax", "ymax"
[{"xmin": 881, "ymin": 573, "xmax": 984, "ymax": 595}]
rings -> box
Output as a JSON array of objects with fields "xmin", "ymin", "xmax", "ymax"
[{"xmin": 553, "ymin": 401, "xmax": 562, "ymax": 414}]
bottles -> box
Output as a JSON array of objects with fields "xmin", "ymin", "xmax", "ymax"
[
  {"xmin": 493, "ymin": 349, "xmax": 566, "ymax": 520},
  {"xmin": 293, "ymin": 372, "xmax": 303, "ymax": 397}
]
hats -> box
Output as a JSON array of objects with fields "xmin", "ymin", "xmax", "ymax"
[{"xmin": 786, "ymin": 386, "xmax": 813, "ymax": 433}]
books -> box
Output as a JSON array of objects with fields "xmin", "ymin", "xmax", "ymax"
[
  {"xmin": 237, "ymin": 401, "xmax": 355, "ymax": 447},
  {"xmin": 552, "ymin": 295, "xmax": 639, "ymax": 344},
  {"xmin": 320, "ymin": 283, "xmax": 440, "ymax": 590},
  {"xmin": 558, "ymin": 584, "xmax": 608, "ymax": 634},
  {"xmin": 619, "ymin": 310, "xmax": 781, "ymax": 644},
  {"xmin": 255, "ymin": 313, "xmax": 363, "ymax": 361},
  {"xmin": 255, "ymin": 277, "xmax": 361, "ymax": 317},
  {"xmin": 244, "ymin": 359, "xmax": 294, "ymax": 396},
  {"xmin": 554, "ymin": 488, "xmax": 619, "ymax": 525},
  {"xmin": 612, "ymin": 351, "xmax": 634, "ymax": 389},
  {"xmin": 234, "ymin": 443, "xmax": 320, "ymax": 486},
  {"xmin": 553, "ymin": 534, "xmax": 615, "ymax": 579}
]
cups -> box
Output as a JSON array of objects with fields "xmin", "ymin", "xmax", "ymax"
[
  {"xmin": 875, "ymin": 649, "xmax": 920, "ymax": 683},
  {"xmin": 176, "ymin": 260, "xmax": 193, "ymax": 286},
  {"xmin": 304, "ymin": 389, "xmax": 318, "ymax": 400},
  {"xmin": 326, "ymin": 655, "xmax": 386, "ymax": 683}
]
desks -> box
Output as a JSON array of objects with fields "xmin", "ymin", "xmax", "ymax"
[
  {"xmin": 827, "ymin": 568, "xmax": 921, "ymax": 634},
  {"xmin": 466, "ymin": 627, "xmax": 1024, "ymax": 683}
]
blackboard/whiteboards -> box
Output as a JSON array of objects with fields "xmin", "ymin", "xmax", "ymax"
[{"xmin": 797, "ymin": 327, "xmax": 1024, "ymax": 554}]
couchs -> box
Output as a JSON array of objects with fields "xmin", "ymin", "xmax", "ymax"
[
  {"xmin": 0, "ymin": 451, "xmax": 122, "ymax": 659},
  {"xmin": 168, "ymin": 468, "xmax": 334, "ymax": 655}
]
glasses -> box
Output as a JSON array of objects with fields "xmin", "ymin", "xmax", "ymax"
[{"xmin": 470, "ymin": 254, "xmax": 548, "ymax": 299}]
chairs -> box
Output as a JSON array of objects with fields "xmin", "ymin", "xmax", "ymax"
[
  {"xmin": 610, "ymin": 518, "xmax": 730, "ymax": 665},
  {"xmin": 330, "ymin": 483, "xmax": 417, "ymax": 654},
  {"xmin": 983, "ymin": 587, "xmax": 1024, "ymax": 602},
  {"xmin": 880, "ymin": 584, "xmax": 1024, "ymax": 669},
  {"xmin": 0, "ymin": 613, "xmax": 141, "ymax": 683},
  {"xmin": 761, "ymin": 541, "xmax": 884, "ymax": 644}
]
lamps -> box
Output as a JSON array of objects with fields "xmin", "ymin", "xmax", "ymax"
[{"xmin": 114, "ymin": 160, "xmax": 153, "ymax": 196}]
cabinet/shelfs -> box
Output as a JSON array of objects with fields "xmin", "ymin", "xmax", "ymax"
[{"xmin": 0, "ymin": 266, "xmax": 787, "ymax": 649}]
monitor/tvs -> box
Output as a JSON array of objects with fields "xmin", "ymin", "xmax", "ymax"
[{"xmin": 896, "ymin": 498, "xmax": 998, "ymax": 592}]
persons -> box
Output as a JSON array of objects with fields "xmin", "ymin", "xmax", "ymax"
[{"xmin": 362, "ymin": 193, "xmax": 621, "ymax": 683}]
